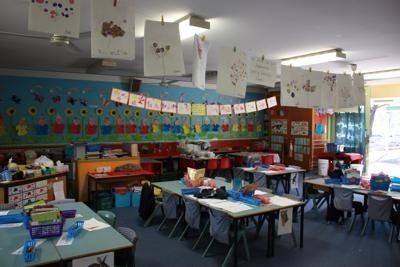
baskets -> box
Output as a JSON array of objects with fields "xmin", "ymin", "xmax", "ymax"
[{"xmin": 29, "ymin": 222, "xmax": 63, "ymax": 238}]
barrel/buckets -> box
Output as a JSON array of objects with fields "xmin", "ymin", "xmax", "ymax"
[
  {"xmin": 350, "ymin": 163, "xmax": 363, "ymax": 178},
  {"xmin": 318, "ymin": 159, "xmax": 329, "ymax": 177}
]
